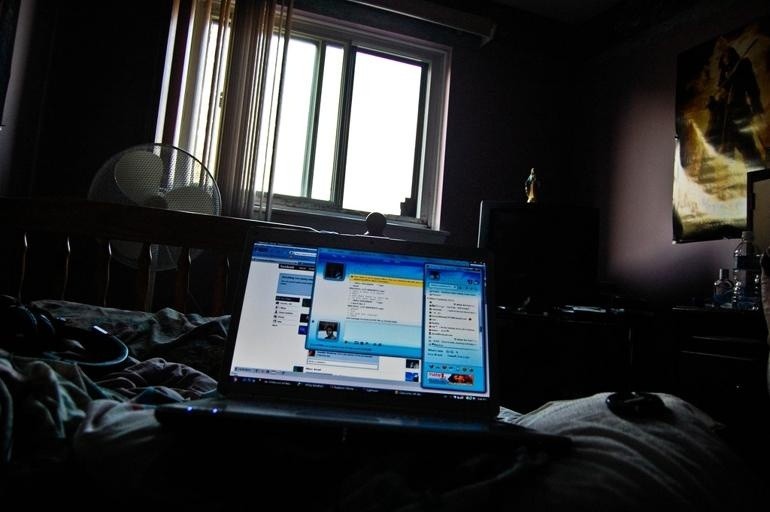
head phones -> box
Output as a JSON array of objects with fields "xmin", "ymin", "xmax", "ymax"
[{"xmin": 0, "ymin": 295, "xmax": 130, "ymax": 377}]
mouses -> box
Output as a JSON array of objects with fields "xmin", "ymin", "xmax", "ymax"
[{"xmin": 607, "ymin": 391, "xmax": 665, "ymax": 423}]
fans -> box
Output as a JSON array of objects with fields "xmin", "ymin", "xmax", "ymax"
[{"xmin": 81, "ymin": 142, "xmax": 225, "ymax": 317}]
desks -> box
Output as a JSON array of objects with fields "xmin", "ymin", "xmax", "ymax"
[{"xmin": 670, "ymin": 298, "xmax": 770, "ymax": 455}]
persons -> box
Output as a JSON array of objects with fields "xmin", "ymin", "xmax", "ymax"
[
  {"xmin": 324, "ymin": 326, "xmax": 336, "ymax": 340},
  {"xmin": 448, "ymin": 374, "xmax": 473, "ymax": 384},
  {"xmin": 703, "ymin": 45, "xmax": 765, "ymax": 201}
]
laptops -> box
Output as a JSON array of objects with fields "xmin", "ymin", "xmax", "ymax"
[{"xmin": 154, "ymin": 226, "xmax": 501, "ymax": 442}]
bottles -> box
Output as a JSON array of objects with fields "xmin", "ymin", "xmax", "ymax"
[{"xmin": 712, "ymin": 231, "xmax": 762, "ymax": 313}]
